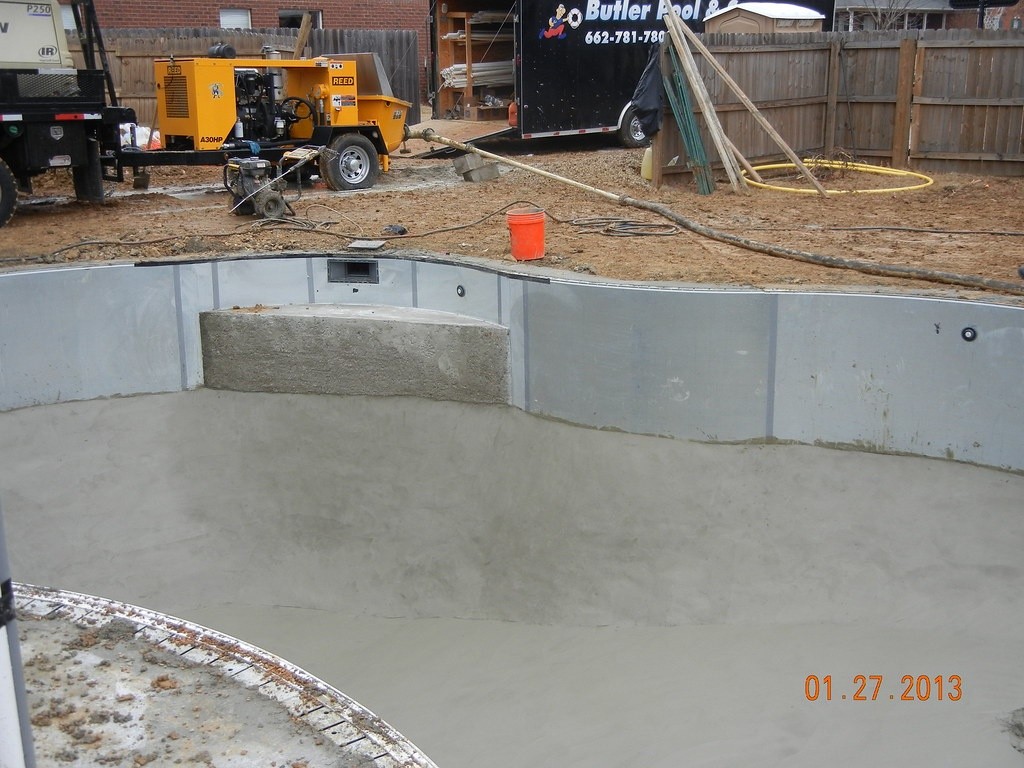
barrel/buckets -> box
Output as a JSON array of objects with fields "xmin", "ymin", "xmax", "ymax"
[{"xmin": 506, "ymin": 208, "xmax": 544, "ymax": 260}]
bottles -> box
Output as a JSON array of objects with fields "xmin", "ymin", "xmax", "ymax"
[
  {"xmin": 509, "ymin": 103, "xmax": 517, "ymax": 126},
  {"xmin": 235, "ymin": 117, "xmax": 243, "ymax": 138},
  {"xmin": 640, "ymin": 140, "xmax": 652, "ymax": 180},
  {"xmin": 326, "ymin": 112, "xmax": 331, "ymax": 126}
]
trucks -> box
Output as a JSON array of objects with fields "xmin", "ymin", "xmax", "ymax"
[{"xmin": 390, "ymin": 0, "xmax": 736, "ymax": 159}]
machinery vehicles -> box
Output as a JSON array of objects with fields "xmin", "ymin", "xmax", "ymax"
[
  {"xmin": 119, "ymin": 41, "xmax": 412, "ymax": 191},
  {"xmin": 0, "ymin": 0, "xmax": 138, "ymax": 228}
]
